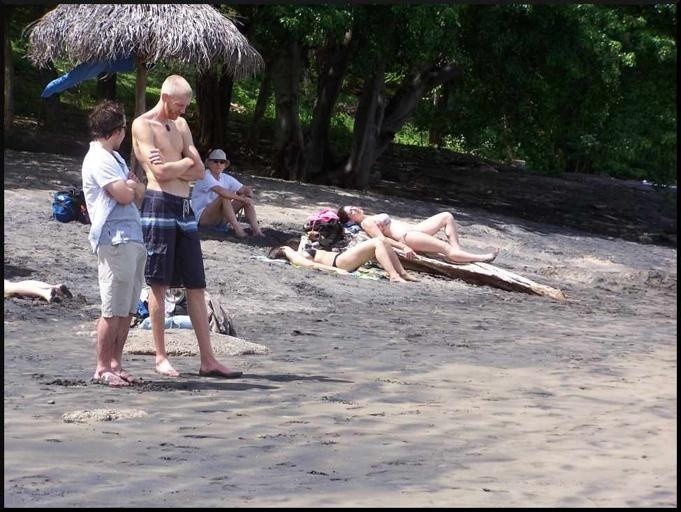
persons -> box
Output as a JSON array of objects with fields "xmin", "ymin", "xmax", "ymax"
[
  {"xmin": 4, "ymin": 278, "xmax": 73, "ymax": 304},
  {"xmin": 479, "ymin": 163, "xmax": 499, "ymax": 173},
  {"xmin": 266, "ymin": 235, "xmax": 422, "ymax": 284},
  {"xmin": 131, "ymin": 75, "xmax": 244, "ymax": 378},
  {"xmin": 339, "ymin": 205, "xmax": 500, "ymax": 264},
  {"xmin": 82, "ymin": 98, "xmax": 145, "ymax": 387},
  {"xmin": 191, "ymin": 149, "xmax": 265, "ymax": 238}
]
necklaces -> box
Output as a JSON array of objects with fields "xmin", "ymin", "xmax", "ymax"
[{"xmin": 157, "ymin": 114, "xmax": 172, "ymax": 132}]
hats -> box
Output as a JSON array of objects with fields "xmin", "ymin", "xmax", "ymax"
[{"xmin": 205, "ymin": 149, "xmax": 230, "ymax": 169}]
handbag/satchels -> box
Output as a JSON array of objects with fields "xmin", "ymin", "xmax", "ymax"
[{"xmin": 53, "ymin": 189, "xmax": 80, "ymax": 222}]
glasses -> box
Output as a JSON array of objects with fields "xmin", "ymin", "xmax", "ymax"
[{"xmin": 214, "ymin": 161, "xmax": 225, "ymax": 163}]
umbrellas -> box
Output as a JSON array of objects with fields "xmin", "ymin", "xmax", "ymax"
[{"xmin": 22, "ymin": 5, "xmax": 268, "ymax": 205}]
